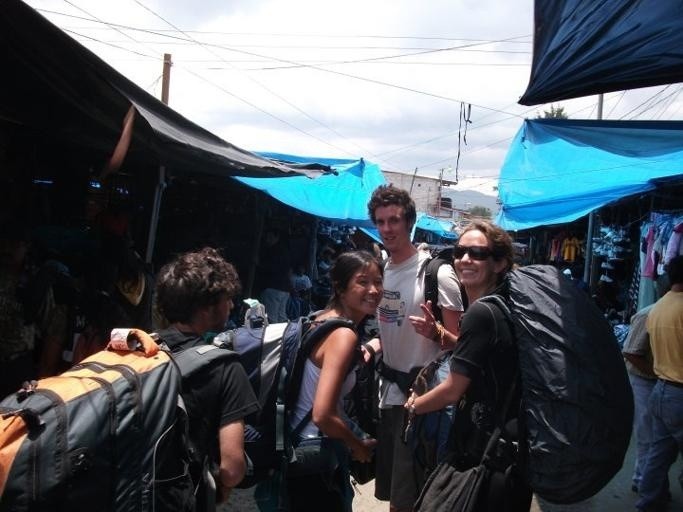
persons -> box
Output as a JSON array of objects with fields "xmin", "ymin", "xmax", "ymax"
[{"xmin": 0, "ymin": 182, "xmax": 681, "ymax": 512}]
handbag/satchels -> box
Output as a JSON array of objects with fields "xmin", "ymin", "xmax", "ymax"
[
  {"xmin": 413, "ymin": 459, "xmax": 488, "ymax": 512},
  {"xmin": 275, "ymin": 445, "xmax": 345, "ymax": 509}
]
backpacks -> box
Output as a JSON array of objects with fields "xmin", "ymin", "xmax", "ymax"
[
  {"xmin": 0, "ymin": 327, "xmax": 244, "ymax": 511},
  {"xmin": 210, "ymin": 299, "xmax": 364, "ymax": 488},
  {"xmin": 475, "ymin": 264, "xmax": 635, "ymax": 505}
]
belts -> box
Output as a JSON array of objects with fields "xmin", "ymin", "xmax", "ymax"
[{"xmin": 659, "ymin": 377, "xmax": 682, "ymax": 389}]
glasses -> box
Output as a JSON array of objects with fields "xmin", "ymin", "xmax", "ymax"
[{"xmin": 452, "ymin": 244, "xmax": 493, "ymax": 262}]
